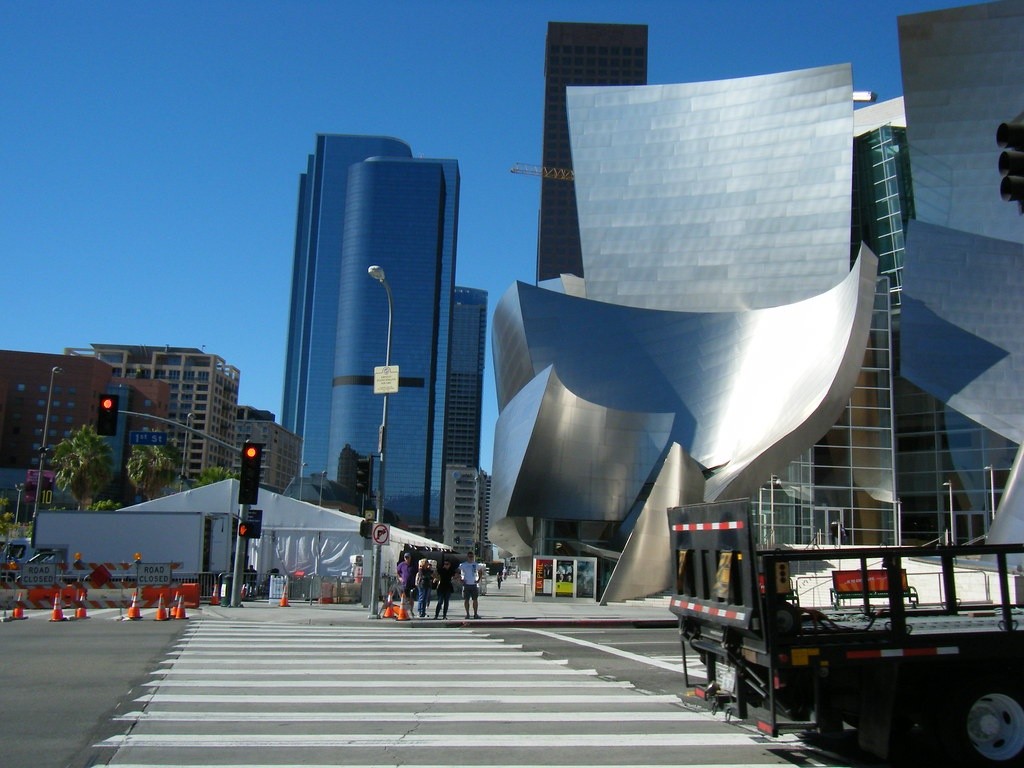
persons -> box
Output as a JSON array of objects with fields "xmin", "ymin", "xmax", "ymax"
[
  {"xmin": 831, "ymin": 522, "xmax": 847, "ymax": 544},
  {"xmin": 455, "ymin": 552, "xmax": 483, "ymax": 619},
  {"xmin": 246, "ymin": 565, "xmax": 258, "ymax": 595},
  {"xmin": 415, "ymin": 559, "xmax": 436, "ymax": 617},
  {"xmin": 502, "ymin": 566, "xmax": 508, "ymax": 580},
  {"xmin": 397, "ymin": 553, "xmax": 416, "ymax": 616},
  {"xmin": 433, "ymin": 561, "xmax": 456, "ymax": 620},
  {"xmin": 497, "ymin": 569, "xmax": 502, "ymax": 589}
]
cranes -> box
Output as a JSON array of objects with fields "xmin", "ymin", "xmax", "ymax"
[{"xmin": 510, "ymin": 161, "xmax": 575, "ymax": 183}]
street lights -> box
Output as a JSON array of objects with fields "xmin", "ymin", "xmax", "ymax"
[
  {"xmin": 319, "ymin": 471, "xmax": 329, "ymax": 506},
  {"xmin": 983, "ymin": 464, "xmax": 996, "ymax": 522},
  {"xmin": 298, "ymin": 462, "xmax": 308, "ymax": 501},
  {"xmin": 13, "ymin": 481, "xmax": 27, "ymax": 524},
  {"xmin": 179, "ymin": 412, "xmax": 196, "ymax": 492},
  {"xmin": 830, "ymin": 520, "xmax": 841, "ymax": 570},
  {"xmin": 943, "ymin": 479, "xmax": 956, "ymax": 547},
  {"xmin": 890, "ymin": 498, "xmax": 903, "ymax": 547},
  {"xmin": 34, "ymin": 365, "xmax": 64, "ymax": 512},
  {"xmin": 367, "ymin": 264, "xmax": 396, "ymax": 618},
  {"xmin": 770, "ymin": 473, "xmax": 783, "ymax": 545}
]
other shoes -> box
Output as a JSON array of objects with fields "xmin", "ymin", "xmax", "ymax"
[
  {"xmin": 465, "ymin": 615, "xmax": 470, "ymax": 619},
  {"xmin": 474, "ymin": 614, "xmax": 481, "ymax": 619},
  {"xmin": 443, "ymin": 616, "xmax": 447, "ymax": 619},
  {"xmin": 419, "ymin": 614, "xmax": 429, "ymax": 617},
  {"xmin": 434, "ymin": 616, "xmax": 437, "ymax": 619}
]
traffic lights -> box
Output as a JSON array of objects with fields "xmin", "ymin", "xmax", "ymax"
[
  {"xmin": 359, "ymin": 520, "xmax": 372, "ymax": 537},
  {"xmin": 995, "ymin": 110, "xmax": 1024, "ymax": 217},
  {"xmin": 356, "ymin": 453, "xmax": 373, "ymax": 501},
  {"xmin": 238, "ymin": 521, "xmax": 253, "ymax": 537},
  {"xmin": 237, "ymin": 442, "xmax": 266, "ymax": 505},
  {"xmin": 97, "ymin": 392, "xmax": 120, "ymax": 437}
]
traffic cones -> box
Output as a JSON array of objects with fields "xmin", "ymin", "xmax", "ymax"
[
  {"xmin": 169, "ymin": 591, "xmax": 181, "ymax": 618},
  {"xmin": 125, "ymin": 591, "xmax": 143, "ymax": 619},
  {"xmin": 278, "ymin": 584, "xmax": 291, "ymax": 608},
  {"xmin": 153, "ymin": 594, "xmax": 170, "ymax": 621},
  {"xmin": 47, "ymin": 593, "xmax": 70, "ymax": 622},
  {"xmin": 208, "ymin": 583, "xmax": 221, "ymax": 605},
  {"xmin": 172, "ymin": 596, "xmax": 189, "ymax": 621},
  {"xmin": 72, "ymin": 592, "xmax": 92, "ymax": 619},
  {"xmin": 9, "ymin": 592, "xmax": 29, "ymax": 620},
  {"xmin": 395, "ymin": 594, "xmax": 409, "ymax": 621},
  {"xmin": 382, "ymin": 591, "xmax": 396, "ymax": 618}
]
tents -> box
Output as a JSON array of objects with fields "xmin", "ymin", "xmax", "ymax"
[{"xmin": 117, "ymin": 478, "xmax": 455, "ymax": 587}]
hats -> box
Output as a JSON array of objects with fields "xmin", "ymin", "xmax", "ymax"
[{"xmin": 404, "ymin": 553, "xmax": 410, "ymax": 558}]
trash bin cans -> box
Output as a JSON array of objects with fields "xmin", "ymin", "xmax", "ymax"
[
  {"xmin": 221, "ymin": 574, "xmax": 233, "ymax": 607},
  {"xmin": 381, "ymin": 575, "xmax": 396, "ymax": 597}
]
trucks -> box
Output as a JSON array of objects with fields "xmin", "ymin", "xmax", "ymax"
[
  {"xmin": 1, "ymin": 509, "xmax": 235, "ymax": 597},
  {"xmin": 666, "ymin": 495, "xmax": 1024, "ymax": 768}
]
